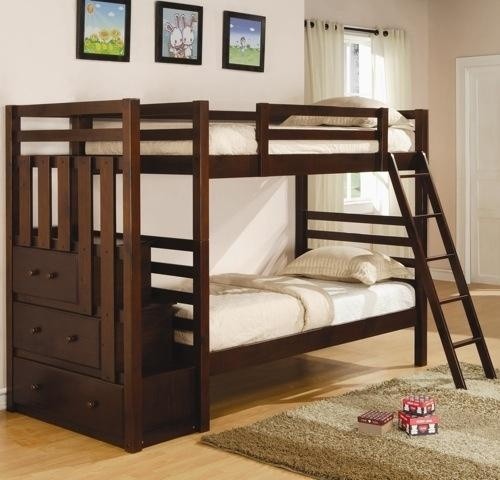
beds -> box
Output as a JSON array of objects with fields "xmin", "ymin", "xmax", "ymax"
[{"xmin": 6, "ymin": 97, "xmax": 497, "ymax": 453}]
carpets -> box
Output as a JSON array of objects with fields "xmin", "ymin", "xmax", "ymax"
[{"xmin": 200, "ymin": 361, "xmax": 498, "ymax": 480}]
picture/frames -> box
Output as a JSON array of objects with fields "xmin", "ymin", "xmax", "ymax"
[{"xmin": 76, "ymin": 0, "xmax": 266, "ymax": 73}]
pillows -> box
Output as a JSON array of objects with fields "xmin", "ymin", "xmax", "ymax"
[
  {"xmin": 277, "ymin": 97, "xmax": 415, "ymax": 131},
  {"xmin": 278, "ymin": 244, "xmax": 414, "ymax": 288}
]
